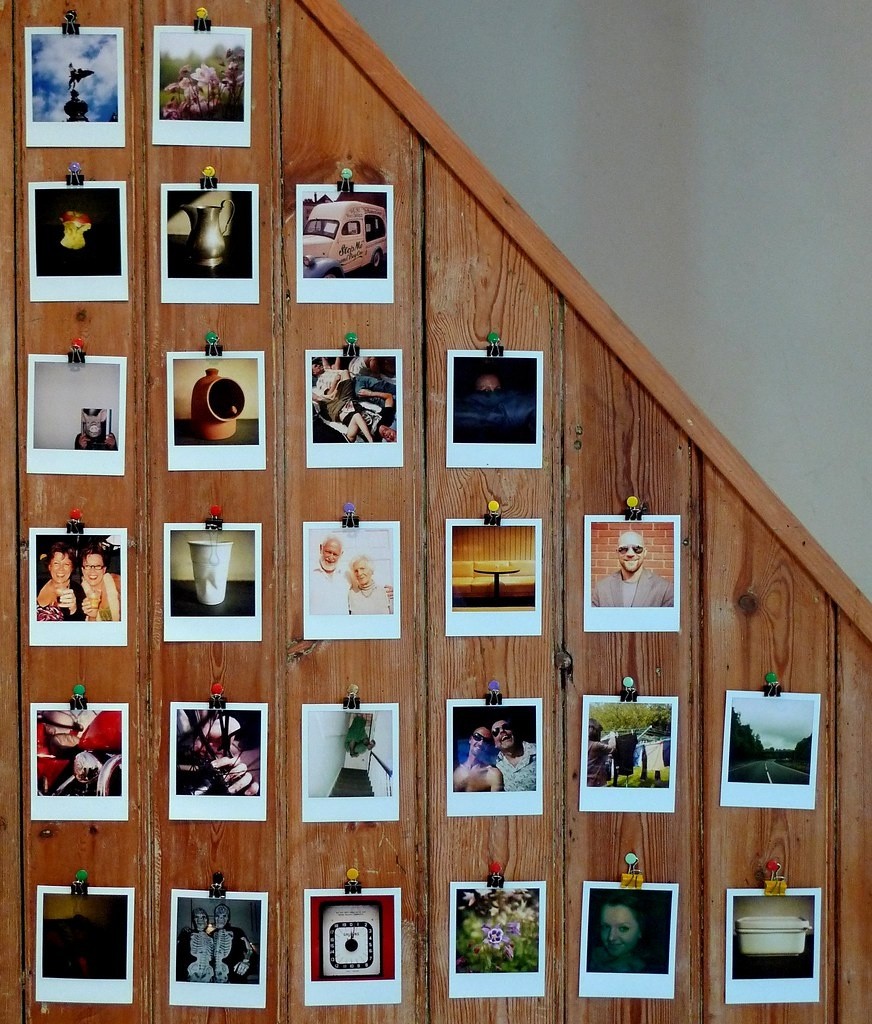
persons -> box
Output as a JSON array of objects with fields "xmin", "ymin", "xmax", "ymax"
[
  {"xmin": 37, "ymin": 541, "xmax": 85, "ymax": 622},
  {"xmin": 588, "ymin": 716, "xmax": 617, "ymax": 787},
  {"xmin": 453, "ymin": 727, "xmax": 504, "ymax": 792},
  {"xmin": 68, "ymin": 62, "xmax": 78, "ymax": 88},
  {"xmin": 491, "ymin": 722, "xmax": 536, "ymax": 791},
  {"xmin": 346, "ymin": 713, "xmax": 374, "ymax": 758},
  {"xmin": 175, "ymin": 710, "xmax": 260, "ymax": 797},
  {"xmin": 599, "ymin": 897, "xmax": 653, "ymax": 968},
  {"xmin": 78, "ymin": 546, "xmax": 121, "ymax": 622},
  {"xmin": 311, "ymin": 356, "xmax": 398, "ymax": 443},
  {"xmin": 591, "ymin": 531, "xmax": 674, "ymax": 607},
  {"xmin": 75, "ymin": 429, "xmax": 118, "ymax": 451},
  {"xmin": 474, "ymin": 373, "xmax": 501, "ymax": 396},
  {"xmin": 308, "ymin": 537, "xmax": 393, "ymax": 613},
  {"xmin": 346, "ymin": 556, "xmax": 393, "ymax": 615}
]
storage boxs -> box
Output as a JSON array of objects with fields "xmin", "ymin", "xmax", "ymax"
[{"xmin": 734, "ymin": 916, "xmax": 812, "ymax": 957}]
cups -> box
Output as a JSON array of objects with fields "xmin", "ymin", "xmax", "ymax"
[
  {"xmin": 88, "ymin": 590, "xmax": 102, "ymax": 610},
  {"xmin": 187, "ymin": 540, "xmax": 235, "ymax": 605},
  {"xmin": 55, "ymin": 584, "xmax": 69, "ymax": 606}
]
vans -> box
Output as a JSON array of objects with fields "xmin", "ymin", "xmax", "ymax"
[{"xmin": 303, "ymin": 201, "xmax": 387, "ymax": 279}]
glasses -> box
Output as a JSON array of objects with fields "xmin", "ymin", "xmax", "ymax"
[
  {"xmin": 617, "ymin": 546, "xmax": 645, "ymax": 554},
  {"xmin": 474, "ymin": 387, "xmax": 503, "ymax": 397},
  {"xmin": 82, "ymin": 565, "xmax": 104, "ymax": 570},
  {"xmin": 492, "ymin": 723, "xmax": 511, "ymax": 737},
  {"xmin": 471, "ymin": 733, "xmax": 489, "ymax": 743}
]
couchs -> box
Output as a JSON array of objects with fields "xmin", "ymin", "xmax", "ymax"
[{"xmin": 452, "ymin": 560, "xmax": 535, "ymax": 599}]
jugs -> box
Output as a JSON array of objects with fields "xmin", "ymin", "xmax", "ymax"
[{"xmin": 181, "ymin": 198, "xmax": 236, "ymax": 267}]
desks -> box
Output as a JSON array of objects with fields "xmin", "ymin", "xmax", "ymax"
[{"xmin": 474, "ymin": 568, "xmax": 519, "ymax": 603}]
